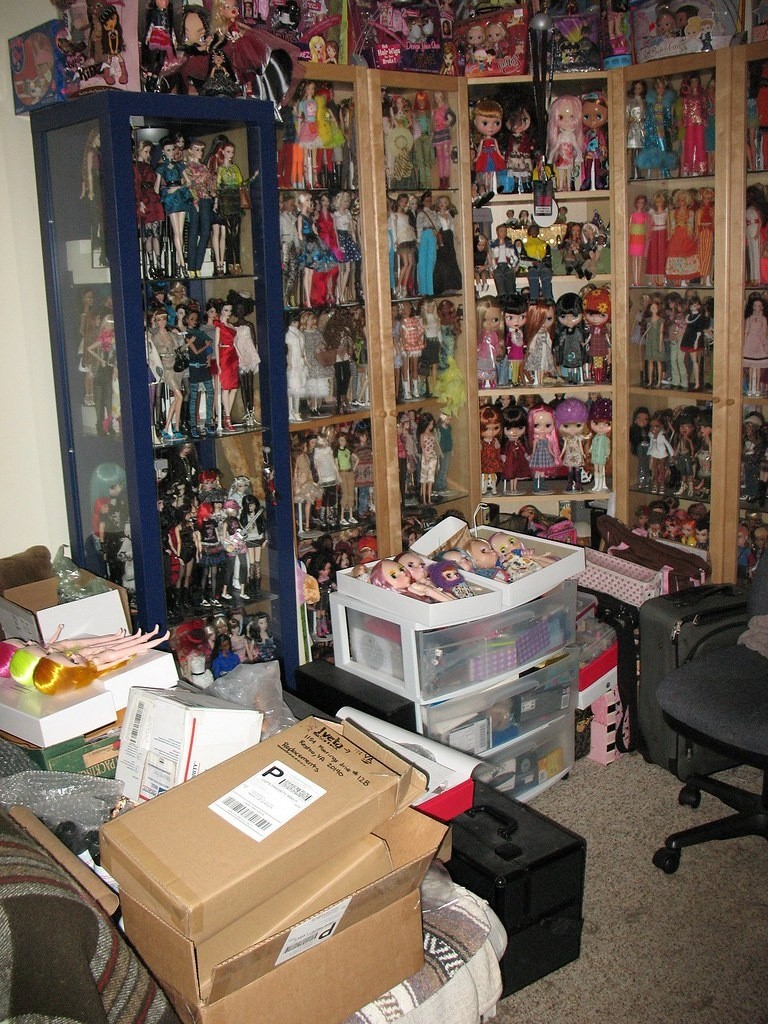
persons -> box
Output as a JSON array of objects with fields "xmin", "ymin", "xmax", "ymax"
[
  {"xmin": 142, "ymin": 0, "xmax": 697, "ymax": 123},
  {"xmin": 90, "ymin": 442, "xmax": 269, "ymax": 614},
  {"xmin": 77, "ymin": 282, "xmax": 260, "ymax": 444},
  {"xmin": 479, "ymin": 392, "xmax": 613, "ymax": 495},
  {"xmin": 383, "ymin": 89, "xmax": 463, "ymax": 300},
  {"xmin": 432, "ymin": 531, "xmax": 559, "ymax": 582},
  {"xmin": 170, "ymin": 611, "xmax": 276, "ymax": 690},
  {"xmin": 353, "ymin": 551, "xmax": 473, "ymax": 603},
  {"xmin": 80, "ymin": 127, "xmax": 259, "ymax": 280},
  {"xmin": 470, "ymin": 92, "xmax": 608, "ymax": 197},
  {"xmin": 519, "ymin": 503, "xmax": 566, "ymax": 533},
  {"xmin": 290, "ymin": 421, "xmax": 377, "ymax": 642},
  {"xmin": 624, "ymin": 72, "xmax": 715, "ymax": 587},
  {"xmin": 25, "ymin": 33, "xmax": 51, "ymax": 102},
  {"xmin": 0, "ymin": 624, "xmax": 170, "ymax": 695},
  {"xmin": 392, "ymin": 299, "xmax": 464, "ymax": 550},
  {"xmin": 475, "ymin": 207, "xmax": 611, "ymax": 388},
  {"xmin": 738, "ymin": 67, "xmax": 768, "ymax": 586},
  {"xmin": 277, "ymin": 81, "xmax": 370, "ymax": 422},
  {"xmin": 69, "ymin": 14, "xmax": 90, "ymax": 45}
]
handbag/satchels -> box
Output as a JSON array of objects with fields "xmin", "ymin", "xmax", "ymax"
[{"xmin": 597, "ymin": 515, "xmax": 711, "ymax": 593}]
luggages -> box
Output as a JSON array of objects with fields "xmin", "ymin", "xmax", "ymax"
[{"xmin": 638, "ymin": 583, "xmax": 753, "ymax": 782}]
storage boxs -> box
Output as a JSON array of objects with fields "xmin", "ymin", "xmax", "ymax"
[
  {"xmin": 6, "ymin": 0, "xmax": 768, "ymax": 116},
  {"xmin": 0, "ymin": 516, "xmax": 588, "ymax": 1024}
]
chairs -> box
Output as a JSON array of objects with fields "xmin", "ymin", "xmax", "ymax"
[{"xmin": 650, "ymin": 546, "xmax": 768, "ymax": 874}]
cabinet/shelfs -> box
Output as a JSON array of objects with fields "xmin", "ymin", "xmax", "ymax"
[{"xmin": 35, "ymin": 46, "xmax": 768, "ymax": 668}]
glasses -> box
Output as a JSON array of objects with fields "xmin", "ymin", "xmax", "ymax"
[{"xmin": 55, "ymin": 821, "xmax": 99, "ymax": 847}]
